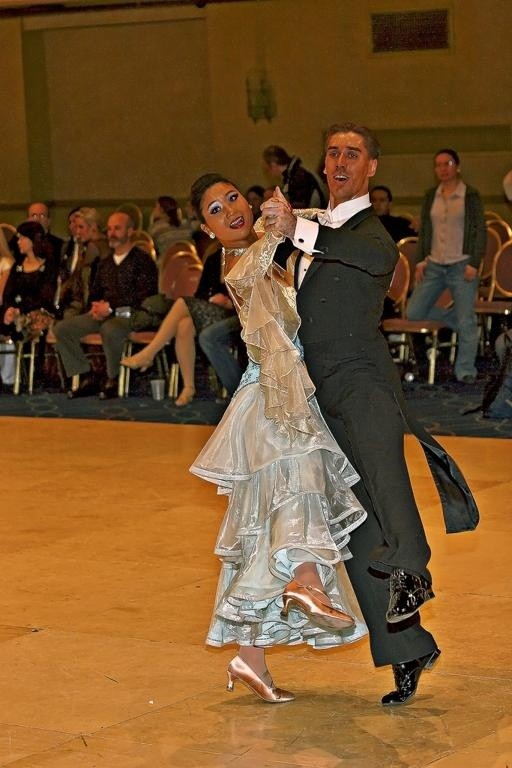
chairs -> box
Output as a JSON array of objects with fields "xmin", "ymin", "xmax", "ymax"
[
  {"xmin": 116, "ymin": 203, "xmax": 143, "ymax": 231},
  {"xmin": 483, "ymin": 224, "xmax": 500, "ymax": 280},
  {"xmin": 118, "ymin": 264, "xmax": 210, "ymax": 401},
  {"xmin": 128, "ymin": 230, "xmax": 154, "ymax": 244},
  {"xmin": 130, "ymin": 240, "xmax": 156, "ymax": 260},
  {"xmin": 484, "ymin": 210, "xmax": 501, "ymax": 222},
  {"xmin": 46, "ymin": 316, "xmax": 103, "ymax": 388},
  {"xmin": 484, "ymin": 218, "xmax": 512, "ymax": 240},
  {"xmin": 161, "ymin": 251, "xmax": 203, "ymax": 297},
  {"xmin": 382, "ymin": 257, "xmax": 477, "ymax": 385},
  {"xmin": 166, "ymin": 238, "xmax": 198, "ymax": 254},
  {"xmin": 473, "ymin": 238, "xmax": 512, "ymax": 318},
  {"xmin": 397, "ymin": 236, "xmax": 419, "ymax": 271},
  {"xmin": 380, "ymin": 248, "xmax": 411, "ymax": 309},
  {"xmin": 5, "ymin": 335, "xmax": 40, "ymax": 395}
]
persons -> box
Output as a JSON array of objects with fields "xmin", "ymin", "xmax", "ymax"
[
  {"xmin": 9, "ymin": 199, "xmax": 64, "ymax": 260},
  {"xmin": 0, "ymin": 228, "xmax": 17, "ymax": 306},
  {"xmin": 258, "ymin": 122, "xmax": 479, "ymax": 708},
  {"xmin": 118, "ymin": 245, "xmax": 234, "ymax": 406},
  {"xmin": 245, "ymin": 185, "xmax": 263, "ymax": 223},
  {"xmin": 147, "ymin": 196, "xmax": 181, "ymax": 238},
  {"xmin": 52, "ymin": 211, "xmax": 158, "ymax": 400},
  {"xmin": 75, "ymin": 205, "xmax": 112, "ymax": 308},
  {"xmin": 198, "ymin": 313, "xmax": 250, "ymax": 406},
  {"xmin": 406, "ymin": 148, "xmax": 486, "ymax": 388},
  {"xmin": 368, "ymin": 185, "xmax": 416, "ymax": 244},
  {"xmin": 261, "ymin": 143, "xmax": 325, "ymax": 214},
  {"xmin": 3, "ymin": 222, "xmax": 61, "ymax": 387},
  {"xmin": 63, "ymin": 207, "xmax": 87, "ymax": 278},
  {"xmin": 186, "ymin": 172, "xmax": 369, "ymax": 706}
]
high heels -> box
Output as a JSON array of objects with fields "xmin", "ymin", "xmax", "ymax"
[
  {"xmin": 176, "ymin": 386, "xmax": 195, "ymax": 406},
  {"xmin": 120, "ymin": 356, "xmax": 154, "ymax": 372},
  {"xmin": 226, "ymin": 656, "xmax": 296, "ymax": 703},
  {"xmin": 280, "ymin": 578, "xmax": 355, "ymax": 630}
]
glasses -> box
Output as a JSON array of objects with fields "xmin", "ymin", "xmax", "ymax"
[
  {"xmin": 435, "ymin": 160, "xmax": 455, "ymax": 167},
  {"xmin": 28, "ymin": 212, "xmax": 47, "ymax": 219}
]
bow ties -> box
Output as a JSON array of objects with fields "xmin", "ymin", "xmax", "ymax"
[{"xmin": 317, "ymin": 208, "xmax": 333, "ymax": 225}]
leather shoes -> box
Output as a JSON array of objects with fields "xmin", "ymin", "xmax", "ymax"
[
  {"xmin": 381, "ymin": 649, "xmax": 441, "ymax": 707},
  {"xmin": 68, "ymin": 381, "xmax": 91, "ymax": 398},
  {"xmin": 98, "ymin": 384, "xmax": 115, "ymax": 399},
  {"xmin": 386, "ymin": 569, "xmax": 436, "ymax": 623},
  {"xmin": 214, "ymin": 396, "xmax": 231, "ymax": 405}
]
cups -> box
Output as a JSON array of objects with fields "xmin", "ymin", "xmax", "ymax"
[{"xmin": 151, "ymin": 380, "xmax": 165, "ymax": 401}]
